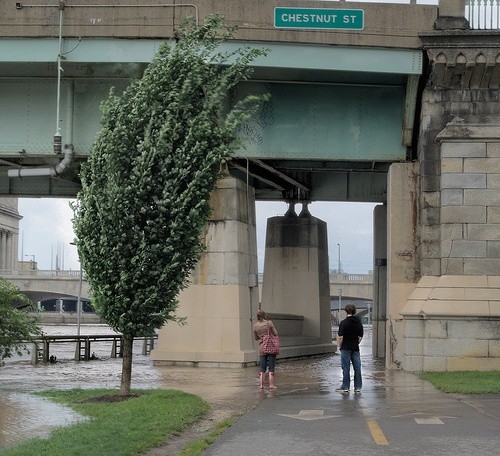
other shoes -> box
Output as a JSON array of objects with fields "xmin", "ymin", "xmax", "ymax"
[
  {"xmin": 336, "ymin": 387, "xmax": 349, "ymax": 392},
  {"xmin": 354, "ymin": 388, "xmax": 360, "ymax": 392}
]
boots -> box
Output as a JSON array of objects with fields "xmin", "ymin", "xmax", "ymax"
[
  {"xmin": 260, "ymin": 372, "xmax": 264, "ymax": 389},
  {"xmin": 268, "ymin": 372, "xmax": 278, "ymax": 389}
]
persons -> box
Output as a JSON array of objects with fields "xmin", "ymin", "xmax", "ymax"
[
  {"xmin": 336, "ymin": 304, "xmax": 364, "ymax": 392},
  {"xmin": 253, "ymin": 311, "xmax": 278, "ymax": 389}
]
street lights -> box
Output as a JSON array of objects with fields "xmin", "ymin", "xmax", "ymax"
[
  {"xmin": 70, "ymin": 242, "xmax": 82, "ymax": 333},
  {"xmin": 367, "ymin": 302, "xmax": 370, "ymax": 342},
  {"xmin": 337, "ymin": 244, "xmax": 341, "ymax": 274},
  {"xmin": 26, "ymin": 254, "xmax": 35, "ymax": 270},
  {"xmin": 338, "ymin": 289, "xmax": 342, "ymax": 324}
]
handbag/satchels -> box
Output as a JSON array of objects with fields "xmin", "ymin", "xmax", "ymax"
[{"xmin": 263, "ymin": 335, "xmax": 279, "ymax": 355}]
